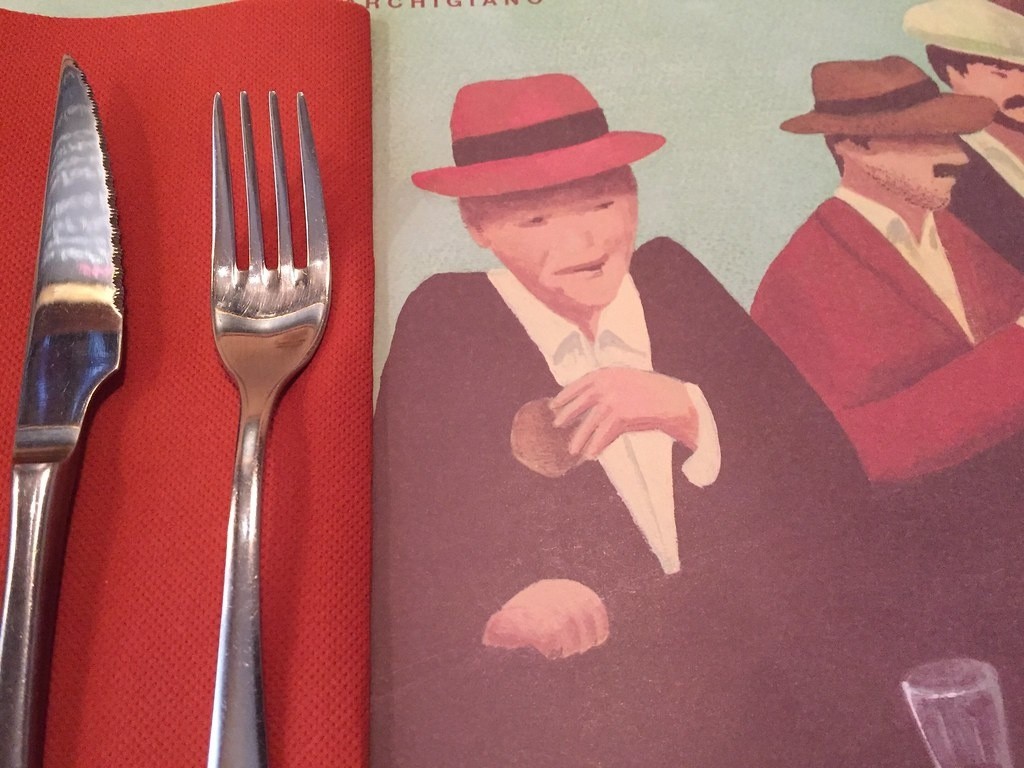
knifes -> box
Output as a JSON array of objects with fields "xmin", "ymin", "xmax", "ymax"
[{"xmin": 0, "ymin": 49, "xmax": 125, "ymax": 768}]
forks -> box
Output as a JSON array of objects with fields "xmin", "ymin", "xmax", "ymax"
[{"xmin": 198, "ymin": 85, "xmax": 334, "ymax": 768}]
